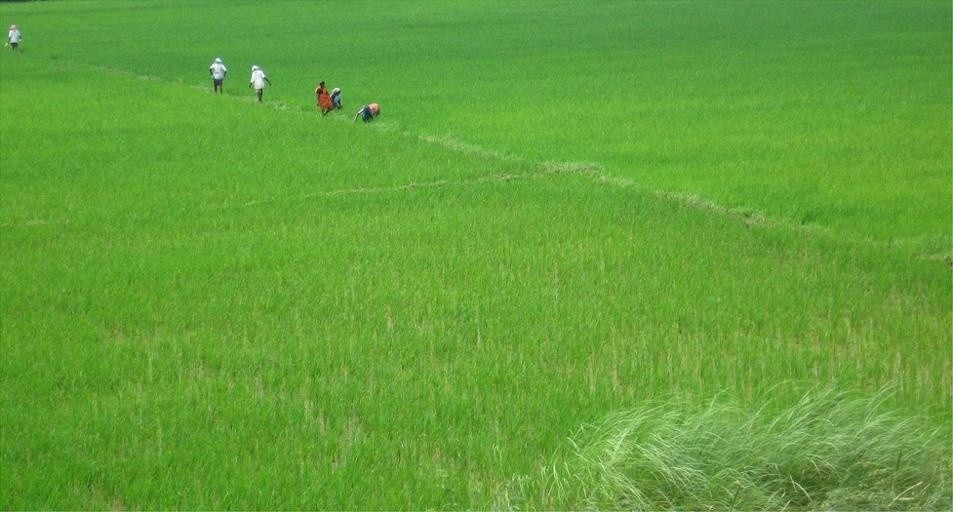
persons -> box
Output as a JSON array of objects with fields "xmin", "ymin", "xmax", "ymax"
[
  {"xmin": 249, "ymin": 64, "xmax": 272, "ymax": 100},
  {"xmin": 330, "ymin": 87, "xmax": 344, "ymax": 109},
  {"xmin": 8, "ymin": 24, "xmax": 22, "ymax": 50},
  {"xmin": 209, "ymin": 56, "xmax": 229, "ymax": 93},
  {"xmin": 315, "ymin": 79, "xmax": 333, "ymax": 116},
  {"xmin": 353, "ymin": 103, "xmax": 382, "ymax": 122}
]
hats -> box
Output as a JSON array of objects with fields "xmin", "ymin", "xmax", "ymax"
[
  {"xmin": 215, "ymin": 58, "xmax": 221, "ymax": 63},
  {"xmin": 333, "ymin": 88, "xmax": 341, "ymax": 95},
  {"xmin": 252, "ymin": 66, "xmax": 260, "ymax": 71},
  {"xmin": 11, "ymin": 25, "xmax": 16, "ymax": 29}
]
